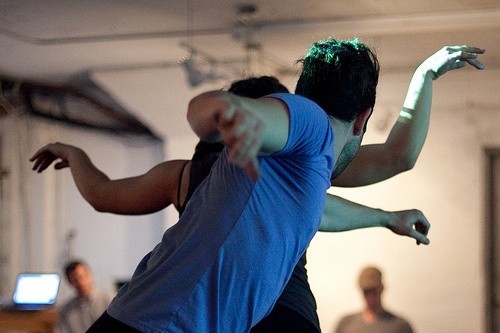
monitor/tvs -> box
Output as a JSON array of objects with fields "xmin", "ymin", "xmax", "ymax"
[{"xmin": 12, "ymin": 273, "xmax": 61, "ymax": 310}]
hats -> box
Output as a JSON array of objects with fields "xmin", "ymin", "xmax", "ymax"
[{"xmin": 360, "ymin": 268, "xmax": 383, "ymax": 289}]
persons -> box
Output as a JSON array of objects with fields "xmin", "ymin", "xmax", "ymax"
[
  {"xmin": 85, "ymin": 35, "xmax": 430, "ymax": 333},
  {"xmin": 51, "ymin": 260, "xmax": 110, "ymax": 333},
  {"xmin": 29, "ymin": 45, "xmax": 486, "ymax": 333},
  {"xmin": 334, "ymin": 267, "xmax": 415, "ymax": 333}
]
